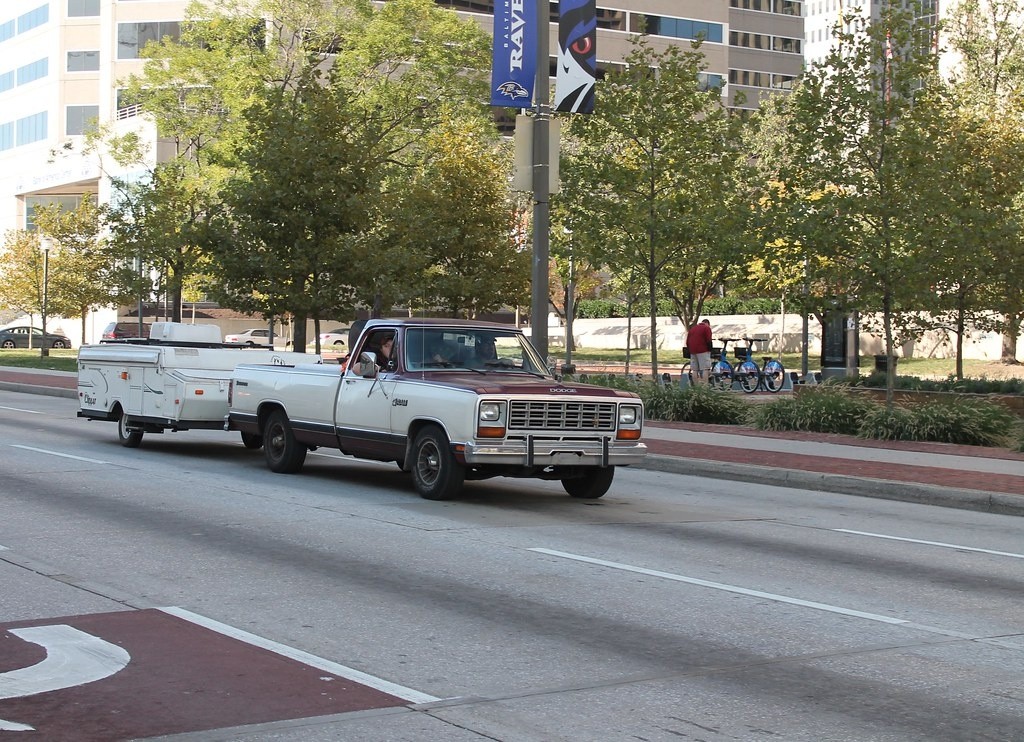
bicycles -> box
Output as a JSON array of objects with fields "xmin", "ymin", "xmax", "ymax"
[
  {"xmin": 680, "ymin": 338, "xmax": 735, "ymax": 392},
  {"xmin": 715, "ymin": 338, "xmax": 760, "ymax": 395},
  {"xmin": 733, "ymin": 337, "xmax": 786, "ymax": 392}
]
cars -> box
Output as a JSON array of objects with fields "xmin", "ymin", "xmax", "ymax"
[
  {"xmin": 315, "ymin": 328, "xmax": 351, "ymax": 346},
  {"xmin": 0, "ymin": 326, "xmax": 72, "ymax": 349},
  {"xmin": 103, "ymin": 322, "xmax": 152, "ymax": 339},
  {"xmin": 225, "ymin": 328, "xmax": 294, "ymax": 347}
]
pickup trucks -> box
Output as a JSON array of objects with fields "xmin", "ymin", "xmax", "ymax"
[{"xmin": 226, "ymin": 314, "xmax": 649, "ymax": 501}]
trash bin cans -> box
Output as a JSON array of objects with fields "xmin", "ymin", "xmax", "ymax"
[{"xmin": 873, "ymin": 354, "xmax": 901, "ymax": 376}]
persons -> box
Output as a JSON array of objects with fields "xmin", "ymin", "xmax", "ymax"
[
  {"xmin": 353, "ymin": 335, "xmax": 394, "ymax": 376},
  {"xmin": 472, "ymin": 334, "xmax": 515, "ymax": 368},
  {"xmin": 687, "ymin": 319, "xmax": 713, "ymax": 383}
]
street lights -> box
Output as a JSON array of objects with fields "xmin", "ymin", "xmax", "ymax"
[{"xmin": 39, "ymin": 235, "xmax": 54, "ymax": 359}]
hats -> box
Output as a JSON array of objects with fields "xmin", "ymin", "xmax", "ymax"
[{"xmin": 381, "ymin": 335, "xmax": 394, "ymax": 345}]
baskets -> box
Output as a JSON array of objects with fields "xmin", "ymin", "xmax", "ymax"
[
  {"xmin": 710, "ymin": 348, "xmax": 722, "ymax": 359},
  {"xmin": 735, "ymin": 347, "xmax": 746, "ymax": 359},
  {"xmin": 682, "ymin": 347, "xmax": 691, "ymax": 358}
]
